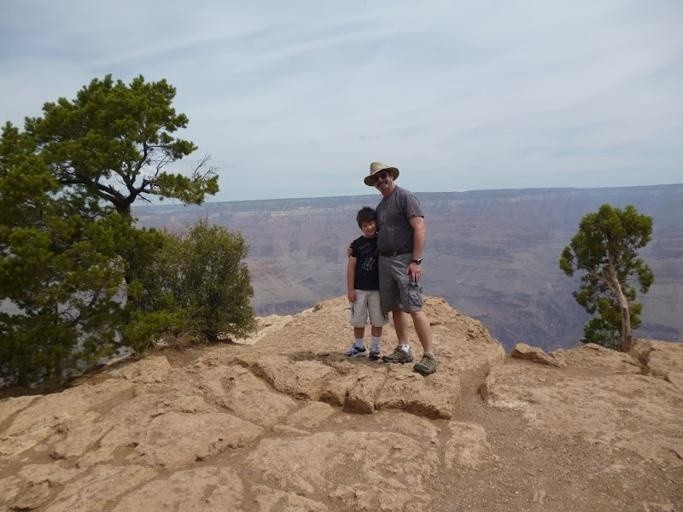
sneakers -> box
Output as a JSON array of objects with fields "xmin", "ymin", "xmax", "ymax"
[
  {"xmin": 414, "ymin": 352, "xmax": 436, "ymax": 373},
  {"xmin": 382, "ymin": 345, "xmax": 413, "ymax": 363},
  {"xmin": 368, "ymin": 349, "xmax": 380, "ymax": 360},
  {"xmin": 343, "ymin": 344, "xmax": 367, "ymax": 358}
]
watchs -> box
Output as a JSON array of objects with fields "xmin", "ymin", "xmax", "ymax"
[{"xmin": 412, "ymin": 257, "xmax": 424, "ymax": 265}]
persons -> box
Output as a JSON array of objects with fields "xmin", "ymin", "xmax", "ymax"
[
  {"xmin": 343, "ymin": 207, "xmax": 383, "ymax": 360},
  {"xmin": 347, "ymin": 160, "xmax": 438, "ymax": 374}
]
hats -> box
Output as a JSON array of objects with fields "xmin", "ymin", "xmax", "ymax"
[{"xmin": 364, "ymin": 162, "xmax": 399, "ymax": 187}]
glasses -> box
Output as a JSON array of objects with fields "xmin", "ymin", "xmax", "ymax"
[{"xmin": 371, "ymin": 172, "xmax": 389, "ymax": 181}]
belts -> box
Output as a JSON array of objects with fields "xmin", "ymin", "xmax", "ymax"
[{"xmin": 378, "ymin": 249, "xmax": 412, "ymax": 255}]
